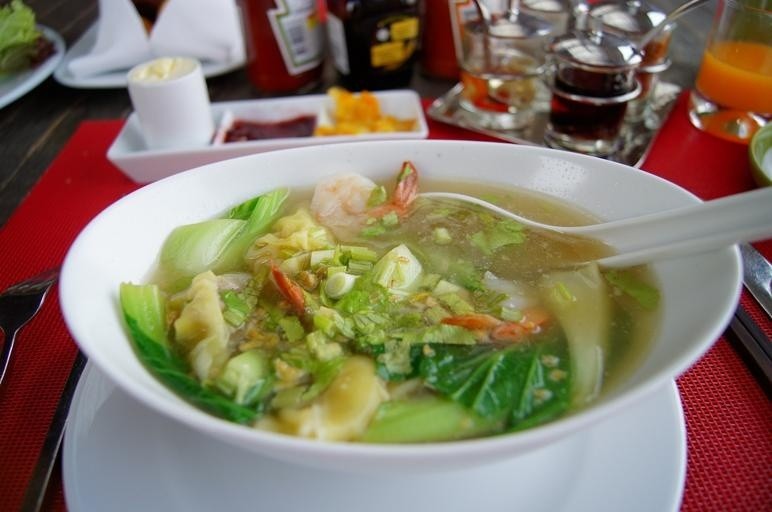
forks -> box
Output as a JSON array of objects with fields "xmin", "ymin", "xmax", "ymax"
[{"xmin": 0, "ymin": 266, "xmax": 63, "ymax": 386}]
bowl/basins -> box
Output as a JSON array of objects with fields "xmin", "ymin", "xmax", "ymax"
[{"xmin": 59, "ymin": 138, "xmax": 745, "ymax": 475}]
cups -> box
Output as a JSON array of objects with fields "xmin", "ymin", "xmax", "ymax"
[
  {"xmin": 438, "ymin": 0, "xmax": 678, "ymax": 150},
  {"xmin": 124, "ymin": 58, "xmax": 216, "ymax": 148}
]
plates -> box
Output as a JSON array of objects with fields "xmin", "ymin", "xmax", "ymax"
[
  {"xmin": 1, "ymin": 22, "xmax": 67, "ymax": 110},
  {"xmin": 61, "ymin": 354, "xmax": 689, "ymax": 512}
]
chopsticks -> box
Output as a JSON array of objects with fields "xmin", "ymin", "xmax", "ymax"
[
  {"xmin": 726, "ymin": 302, "xmax": 772, "ymax": 400},
  {"xmin": 20, "ymin": 349, "xmax": 87, "ymax": 506}
]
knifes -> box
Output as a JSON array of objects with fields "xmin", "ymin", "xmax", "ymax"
[{"xmin": 742, "ymin": 239, "xmax": 769, "ymax": 316}]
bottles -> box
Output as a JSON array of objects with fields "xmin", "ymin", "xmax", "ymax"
[
  {"xmin": 235, "ymin": 0, "xmax": 324, "ymax": 94},
  {"xmin": 326, "ymin": 0, "xmax": 427, "ymax": 96}
]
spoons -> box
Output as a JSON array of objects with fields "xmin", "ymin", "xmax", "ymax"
[{"xmin": 410, "ymin": 185, "xmax": 772, "ymax": 272}]
